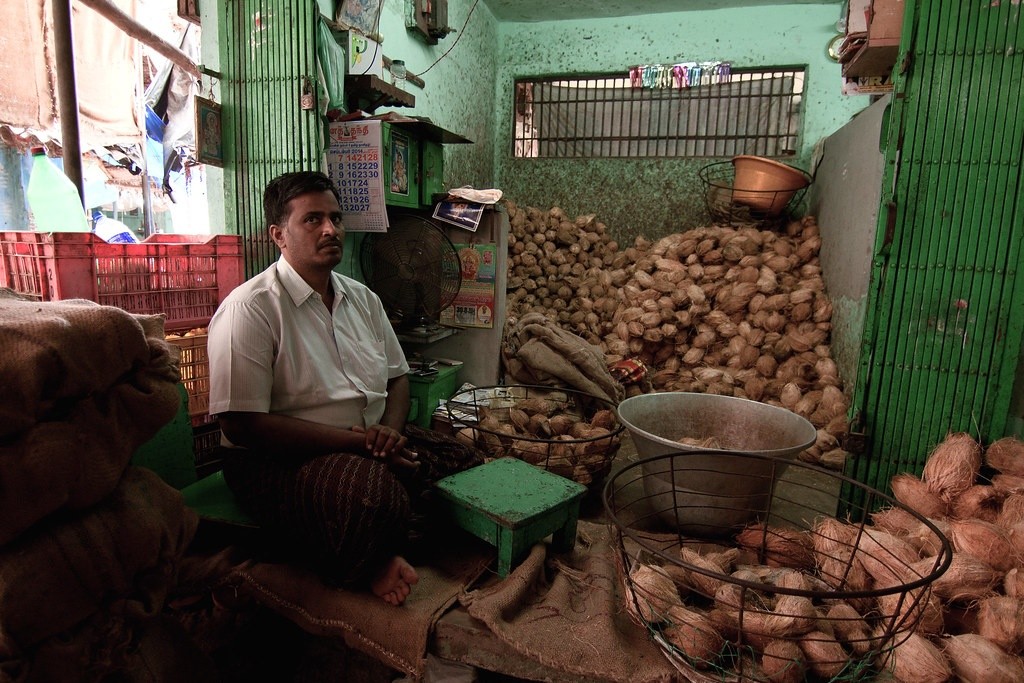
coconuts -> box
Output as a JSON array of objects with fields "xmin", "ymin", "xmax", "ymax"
[
  {"xmin": 447, "ymin": 198, "xmax": 847, "ymax": 488},
  {"xmin": 625, "ymin": 431, "xmax": 1024, "ymax": 683}
]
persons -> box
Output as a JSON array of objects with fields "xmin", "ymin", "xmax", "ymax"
[{"xmin": 207, "ymin": 170, "xmax": 485, "ymax": 605}]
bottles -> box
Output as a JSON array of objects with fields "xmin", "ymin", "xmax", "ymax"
[
  {"xmin": 390, "ymin": 60, "xmax": 406, "ymax": 92},
  {"xmin": 92, "ymin": 211, "xmax": 154, "ymax": 272},
  {"xmin": 27, "ymin": 147, "xmax": 90, "ymax": 233}
]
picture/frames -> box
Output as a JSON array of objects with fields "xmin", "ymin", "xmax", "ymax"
[{"xmin": 193, "ymin": 95, "xmax": 225, "ymax": 169}]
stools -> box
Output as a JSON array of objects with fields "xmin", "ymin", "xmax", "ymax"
[{"xmin": 435, "ymin": 456, "xmax": 586, "ymax": 579}]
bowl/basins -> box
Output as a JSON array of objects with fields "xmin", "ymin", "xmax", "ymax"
[{"xmin": 731, "ymin": 155, "xmax": 810, "ymax": 216}]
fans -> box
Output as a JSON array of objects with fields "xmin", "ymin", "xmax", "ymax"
[{"xmin": 358, "ymin": 214, "xmax": 465, "ymax": 337}]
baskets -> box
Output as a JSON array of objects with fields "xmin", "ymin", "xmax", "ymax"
[
  {"xmin": 698, "ymin": 159, "xmax": 814, "ymax": 226},
  {"xmin": 602, "ymin": 450, "xmax": 953, "ymax": 683},
  {"xmin": 446, "ymin": 384, "xmax": 626, "ymax": 484}
]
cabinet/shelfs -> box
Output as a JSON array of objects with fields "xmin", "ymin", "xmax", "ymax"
[{"xmin": 358, "ymin": 110, "xmax": 444, "ymax": 207}]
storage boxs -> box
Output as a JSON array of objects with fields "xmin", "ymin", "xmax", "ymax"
[
  {"xmin": 842, "ymin": 0, "xmax": 905, "ymax": 77},
  {"xmin": 0, "ymin": 232, "xmax": 244, "ymax": 331},
  {"xmin": 407, "ymin": 363, "xmax": 463, "ymax": 428},
  {"xmin": 166, "ymin": 334, "xmax": 211, "ymax": 427}
]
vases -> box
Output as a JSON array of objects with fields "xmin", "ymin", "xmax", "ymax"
[{"xmin": 730, "ymin": 155, "xmax": 810, "ymax": 214}]
